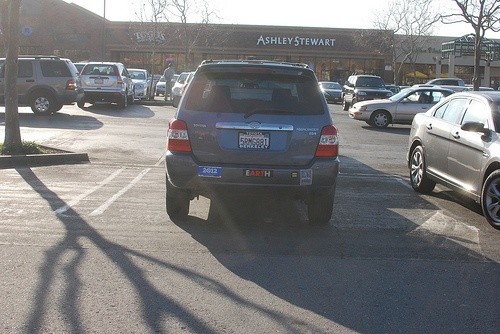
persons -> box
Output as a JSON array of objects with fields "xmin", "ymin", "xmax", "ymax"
[
  {"xmin": 420, "ymin": 94, "xmax": 427, "ymax": 103},
  {"xmin": 163, "ymin": 63, "xmax": 173, "ymax": 101}
]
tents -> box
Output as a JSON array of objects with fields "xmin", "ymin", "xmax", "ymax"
[
  {"xmin": 436, "ymin": 72, "xmax": 448, "ymax": 77},
  {"xmin": 406, "ymin": 71, "xmax": 427, "ymax": 78}
]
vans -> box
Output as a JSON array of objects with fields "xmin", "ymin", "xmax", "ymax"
[{"xmin": 427, "ymin": 77, "xmax": 465, "ymax": 85}]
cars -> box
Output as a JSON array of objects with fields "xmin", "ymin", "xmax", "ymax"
[
  {"xmin": 350, "ymin": 84, "xmax": 457, "ymax": 130},
  {"xmin": 171, "ymin": 71, "xmax": 194, "ymax": 106},
  {"xmin": 155, "ymin": 74, "xmax": 181, "ymax": 95},
  {"xmin": 318, "ymin": 80, "xmax": 342, "ymax": 103},
  {"xmin": 405, "ymin": 89, "xmax": 500, "ymax": 229}
]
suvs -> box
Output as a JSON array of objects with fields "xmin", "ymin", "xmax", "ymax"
[
  {"xmin": 0, "ymin": 54, "xmax": 85, "ymax": 116},
  {"xmin": 340, "ymin": 71, "xmax": 394, "ymax": 111},
  {"xmin": 126, "ymin": 68, "xmax": 156, "ymax": 101},
  {"xmin": 76, "ymin": 59, "xmax": 134, "ymax": 108},
  {"xmin": 165, "ymin": 57, "xmax": 339, "ymax": 223}
]
